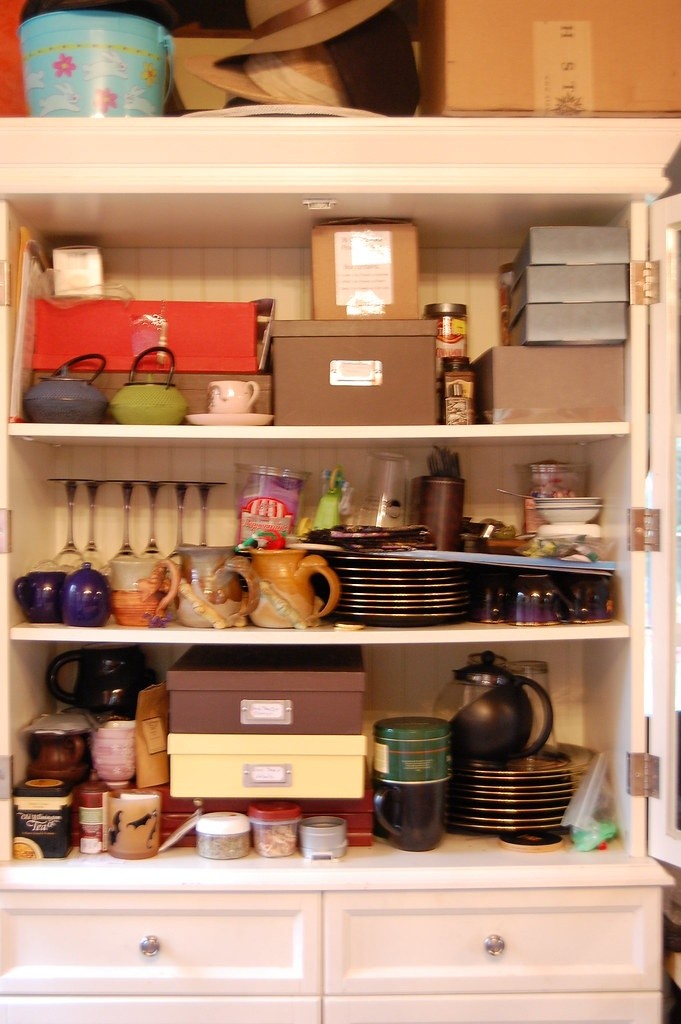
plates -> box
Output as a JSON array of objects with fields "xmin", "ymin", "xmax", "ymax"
[
  {"xmin": 445, "ymin": 750, "xmax": 597, "ymax": 835},
  {"xmin": 184, "ymin": 412, "xmax": 275, "ymax": 427},
  {"xmin": 317, "ymin": 552, "xmax": 473, "ymax": 628}
]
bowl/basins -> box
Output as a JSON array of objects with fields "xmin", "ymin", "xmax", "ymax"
[{"xmin": 533, "ymin": 497, "xmax": 603, "ymax": 525}]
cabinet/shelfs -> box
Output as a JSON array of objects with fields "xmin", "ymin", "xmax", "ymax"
[{"xmin": 1, "ymin": 113, "xmax": 679, "ymax": 866}]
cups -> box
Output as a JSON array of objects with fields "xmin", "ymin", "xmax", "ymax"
[
  {"xmin": 417, "ymin": 475, "xmax": 466, "ymax": 551},
  {"xmin": 507, "ymin": 658, "xmax": 558, "ymax": 767},
  {"xmin": 90, "ymin": 720, "xmax": 136, "ymax": 786},
  {"xmin": 207, "ymin": 380, "xmax": 260, "ymax": 414},
  {"xmin": 470, "ymin": 564, "xmax": 614, "ymax": 626},
  {"xmin": 373, "ymin": 774, "xmax": 453, "ymax": 852},
  {"xmin": 13, "ymin": 544, "xmax": 341, "ymax": 629},
  {"xmin": 351, "ymin": 448, "xmax": 411, "ymax": 529},
  {"xmin": 106, "ymin": 789, "xmax": 162, "ymax": 860}
]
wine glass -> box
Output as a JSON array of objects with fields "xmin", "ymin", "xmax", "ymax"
[{"xmin": 47, "ymin": 478, "xmax": 228, "ymax": 580}]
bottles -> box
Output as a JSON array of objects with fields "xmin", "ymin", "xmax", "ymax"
[
  {"xmin": 422, "ymin": 303, "xmax": 468, "ymax": 380},
  {"xmin": 440, "ymin": 356, "xmax": 476, "ymax": 425}
]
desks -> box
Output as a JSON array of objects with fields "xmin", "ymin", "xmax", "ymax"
[{"xmin": 4, "ymin": 867, "xmax": 681, "ymax": 1024}]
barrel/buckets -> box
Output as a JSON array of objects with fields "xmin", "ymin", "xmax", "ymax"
[{"xmin": 17, "ymin": 10, "xmax": 174, "ymax": 117}]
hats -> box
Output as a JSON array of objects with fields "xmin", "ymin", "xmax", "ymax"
[{"xmin": 168, "ymin": 0, "xmax": 422, "ymax": 121}]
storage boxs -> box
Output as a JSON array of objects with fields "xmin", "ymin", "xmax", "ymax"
[
  {"xmin": 16, "ymin": 220, "xmax": 627, "ymax": 422},
  {"xmin": 80, "ymin": 650, "xmax": 376, "ymax": 845},
  {"xmin": 420, "ymin": 1, "xmax": 681, "ymax": 119}
]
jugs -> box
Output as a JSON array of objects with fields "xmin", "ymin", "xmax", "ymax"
[
  {"xmin": 108, "ymin": 346, "xmax": 189, "ymax": 425},
  {"xmin": 45, "ymin": 642, "xmax": 158, "ymax": 721},
  {"xmin": 432, "ymin": 650, "xmax": 554, "ymax": 767},
  {"xmin": 22, "ymin": 352, "xmax": 109, "ymax": 423}
]
